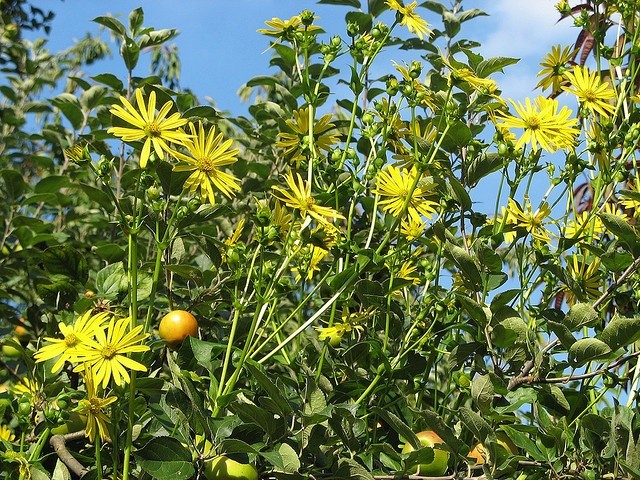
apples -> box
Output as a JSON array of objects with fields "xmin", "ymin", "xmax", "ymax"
[
  {"xmin": 2, "ymin": 338, "xmax": 23, "ymax": 358},
  {"xmin": 15, "ymin": 317, "xmax": 32, "ymax": 342},
  {"xmin": 179, "ymin": 434, "xmax": 216, "ymax": 461},
  {"xmin": 401, "ymin": 430, "xmax": 450, "ymax": 476},
  {"xmin": 159, "ymin": 310, "xmax": 199, "ymax": 350},
  {"xmin": 204, "ymin": 454, "xmax": 258, "ymax": 479},
  {"xmin": 467, "ymin": 444, "xmax": 487, "ymax": 466},
  {"xmin": 495, "ymin": 433, "xmax": 519, "ymax": 454}
]
